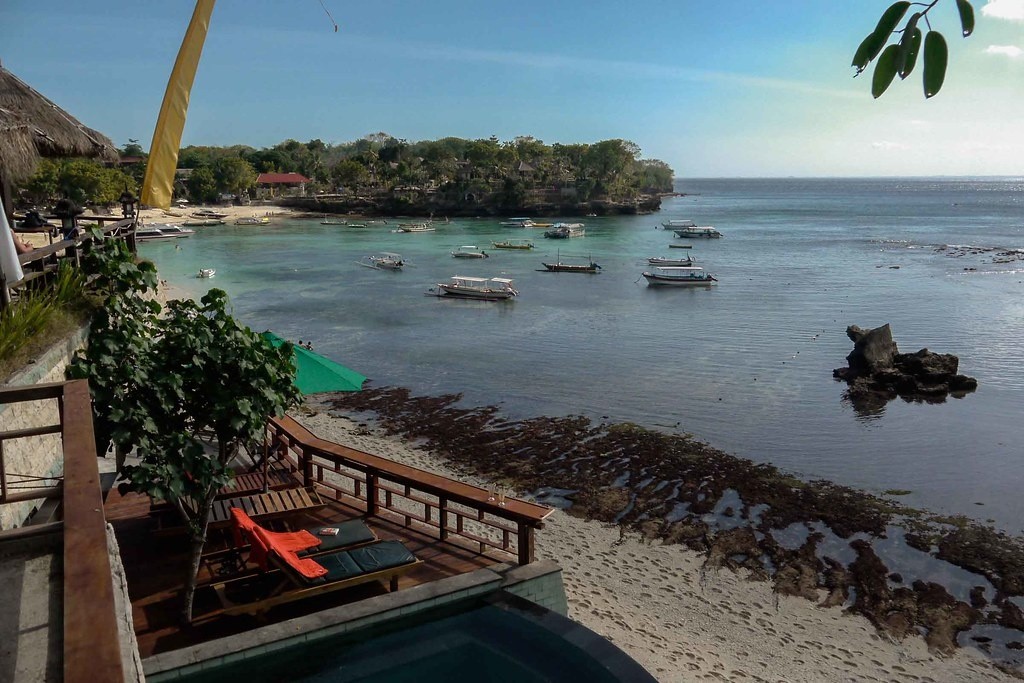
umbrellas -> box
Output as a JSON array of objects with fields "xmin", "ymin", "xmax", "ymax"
[{"xmin": 262, "ymin": 330, "xmax": 367, "ymax": 492}]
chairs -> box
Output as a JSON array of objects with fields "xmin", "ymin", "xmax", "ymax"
[{"xmin": 162, "ymin": 468, "xmax": 424, "ymax": 615}]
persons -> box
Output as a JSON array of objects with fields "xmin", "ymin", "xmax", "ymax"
[
  {"xmin": 297, "ymin": 340, "xmax": 306, "ymax": 349},
  {"xmin": 306, "ymin": 341, "xmax": 313, "ymax": 350},
  {"xmin": 11, "ymin": 228, "xmax": 43, "ymax": 272}
]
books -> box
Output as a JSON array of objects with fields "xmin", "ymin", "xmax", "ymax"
[{"xmin": 319, "ymin": 528, "xmax": 339, "ymax": 535}]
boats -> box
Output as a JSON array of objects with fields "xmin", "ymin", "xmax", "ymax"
[
  {"xmin": 542, "ymin": 262, "xmax": 597, "ymax": 273},
  {"xmin": 348, "ymin": 224, "xmax": 367, "ymax": 228},
  {"xmin": 369, "ymin": 256, "xmax": 406, "ymax": 268},
  {"xmin": 235, "ymin": 218, "xmax": 271, "ymax": 226},
  {"xmin": 136, "ymin": 223, "xmax": 196, "ymax": 241},
  {"xmin": 424, "ymin": 219, "xmax": 452, "ymax": 224},
  {"xmin": 646, "ymin": 258, "xmax": 693, "ymax": 266},
  {"xmin": 586, "ymin": 213, "xmax": 597, "ymax": 217},
  {"xmin": 661, "ymin": 220, "xmax": 697, "ymax": 230},
  {"xmin": 197, "ymin": 268, "xmax": 217, "ymax": 277},
  {"xmin": 437, "ymin": 276, "xmax": 520, "ymax": 299},
  {"xmin": 391, "ymin": 224, "xmax": 435, "ymax": 232},
  {"xmin": 452, "ymin": 250, "xmax": 489, "ymax": 258},
  {"xmin": 499, "ymin": 217, "xmax": 554, "ymax": 227},
  {"xmin": 544, "ymin": 223, "xmax": 585, "ymax": 239},
  {"xmin": 366, "ymin": 220, "xmax": 388, "ymax": 224},
  {"xmin": 321, "ymin": 213, "xmax": 347, "ymax": 225},
  {"xmin": 189, "ymin": 209, "xmax": 229, "ymax": 218},
  {"xmin": 491, "ymin": 239, "xmax": 536, "ymax": 248},
  {"xmin": 643, "ymin": 267, "xmax": 717, "ymax": 286},
  {"xmin": 165, "ymin": 210, "xmax": 184, "ymax": 217},
  {"xmin": 675, "ymin": 227, "xmax": 723, "ymax": 238},
  {"xmin": 182, "ymin": 220, "xmax": 227, "ymax": 226}
]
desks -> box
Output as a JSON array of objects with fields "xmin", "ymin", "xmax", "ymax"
[{"xmin": 14, "ymin": 225, "xmax": 57, "ymax": 245}]
